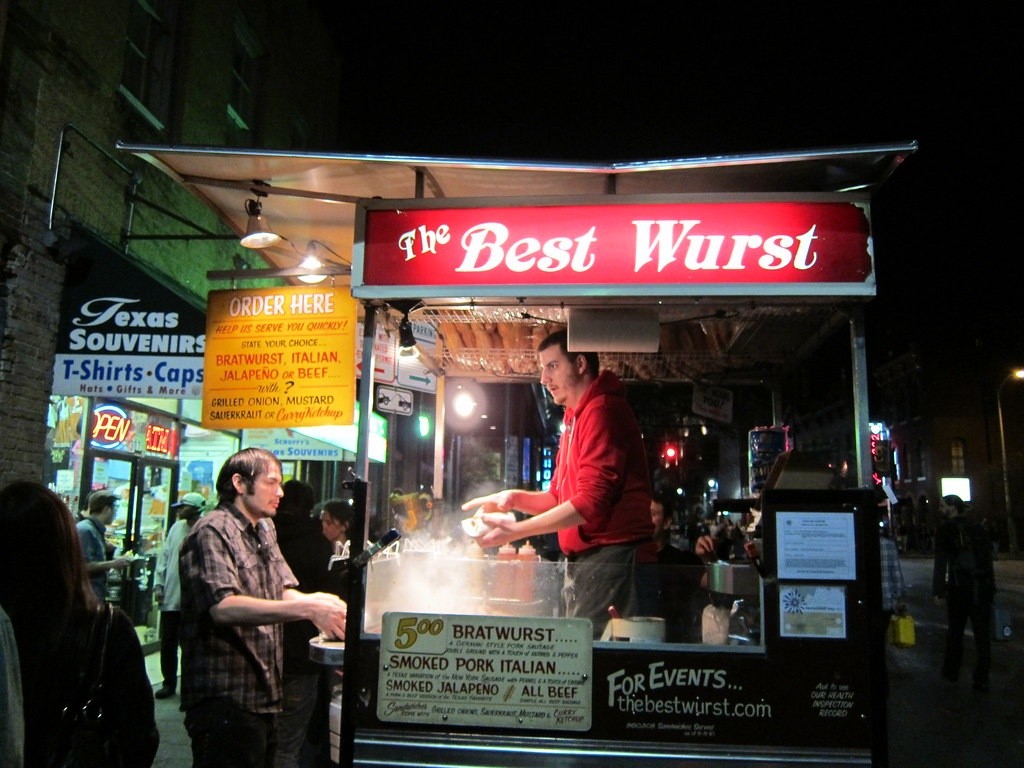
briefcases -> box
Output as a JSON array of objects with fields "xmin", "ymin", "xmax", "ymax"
[
  {"xmin": 888, "ymin": 611, "xmax": 916, "ymax": 647},
  {"xmin": 991, "ymin": 601, "xmax": 1014, "ymax": 641}
]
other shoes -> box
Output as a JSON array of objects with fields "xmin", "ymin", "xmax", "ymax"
[{"xmin": 156, "ymin": 685, "xmax": 176, "ymax": 699}]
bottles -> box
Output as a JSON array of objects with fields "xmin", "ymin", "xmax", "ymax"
[
  {"xmin": 493, "ymin": 544, "xmax": 515, "ymax": 601},
  {"xmin": 515, "ymin": 540, "xmax": 540, "ymax": 600}
]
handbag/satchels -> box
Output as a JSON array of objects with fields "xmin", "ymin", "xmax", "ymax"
[{"xmin": 48, "ymin": 603, "xmax": 124, "ymax": 768}]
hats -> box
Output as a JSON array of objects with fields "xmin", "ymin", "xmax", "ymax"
[
  {"xmin": 89, "ymin": 492, "xmax": 121, "ymax": 511},
  {"xmin": 171, "ymin": 492, "xmax": 205, "ymax": 507}
]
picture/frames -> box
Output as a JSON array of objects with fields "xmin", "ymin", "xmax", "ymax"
[{"xmin": 349, "ymin": 191, "xmax": 874, "ymax": 296}]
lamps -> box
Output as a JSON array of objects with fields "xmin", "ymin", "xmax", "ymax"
[
  {"xmin": 296, "ymin": 239, "xmax": 328, "ymax": 283},
  {"xmin": 241, "ymin": 199, "xmax": 281, "ymax": 250},
  {"xmin": 385, "ymin": 299, "xmax": 426, "ymax": 360}
]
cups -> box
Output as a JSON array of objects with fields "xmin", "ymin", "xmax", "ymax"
[{"xmin": 392, "ymin": 491, "xmax": 434, "ymax": 533}]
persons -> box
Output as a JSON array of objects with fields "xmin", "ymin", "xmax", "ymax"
[
  {"xmin": 0, "ymin": 481, "xmax": 161, "ymax": 768},
  {"xmin": 679, "ymin": 507, "xmax": 757, "ymax": 561},
  {"xmin": 269, "ymin": 478, "xmax": 354, "ymax": 768},
  {"xmin": 460, "ymin": 328, "xmax": 651, "ymax": 641},
  {"xmin": 71, "ymin": 486, "xmax": 134, "ymax": 604},
  {"xmin": 152, "ymin": 491, "xmax": 205, "ymax": 711},
  {"xmin": 176, "ymin": 448, "xmax": 348, "ymax": 768},
  {"xmin": 879, "ymin": 536, "xmax": 906, "ymax": 637},
  {"xmin": 632, "ymin": 492, "xmax": 716, "ymax": 643},
  {"xmin": 932, "ymin": 495, "xmax": 998, "ymax": 698}
]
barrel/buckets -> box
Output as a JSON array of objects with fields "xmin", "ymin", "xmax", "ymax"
[{"xmin": 611, "ymin": 616, "xmax": 667, "ymax": 644}]
